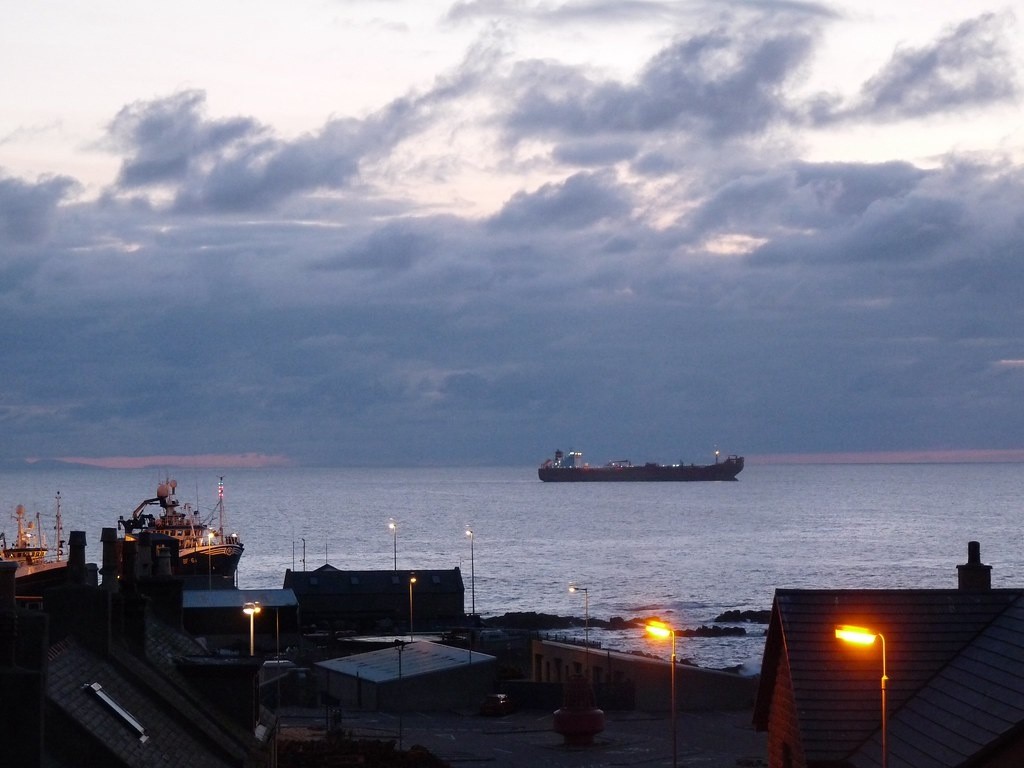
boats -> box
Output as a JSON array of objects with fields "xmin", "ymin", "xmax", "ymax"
[
  {"xmin": 116, "ymin": 473, "xmax": 245, "ymax": 573},
  {"xmin": 0, "ymin": 488, "xmax": 66, "ymax": 578},
  {"xmin": 537, "ymin": 444, "xmax": 745, "ymax": 484}
]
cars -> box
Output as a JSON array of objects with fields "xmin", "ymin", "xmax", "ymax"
[{"xmin": 483, "ymin": 695, "xmax": 517, "ymax": 716}]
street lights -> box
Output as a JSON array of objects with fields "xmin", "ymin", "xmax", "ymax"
[
  {"xmin": 241, "ymin": 601, "xmax": 261, "ymax": 656},
  {"xmin": 406, "ymin": 571, "xmax": 418, "ymax": 643},
  {"xmin": 567, "ymin": 579, "xmax": 589, "ymax": 652},
  {"xmin": 831, "ymin": 619, "xmax": 890, "ymax": 768},
  {"xmin": 641, "ymin": 613, "xmax": 679, "ymax": 768},
  {"xmin": 387, "ymin": 515, "xmax": 400, "ymax": 571},
  {"xmin": 466, "ymin": 528, "xmax": 477, "ymax": 617}
]
